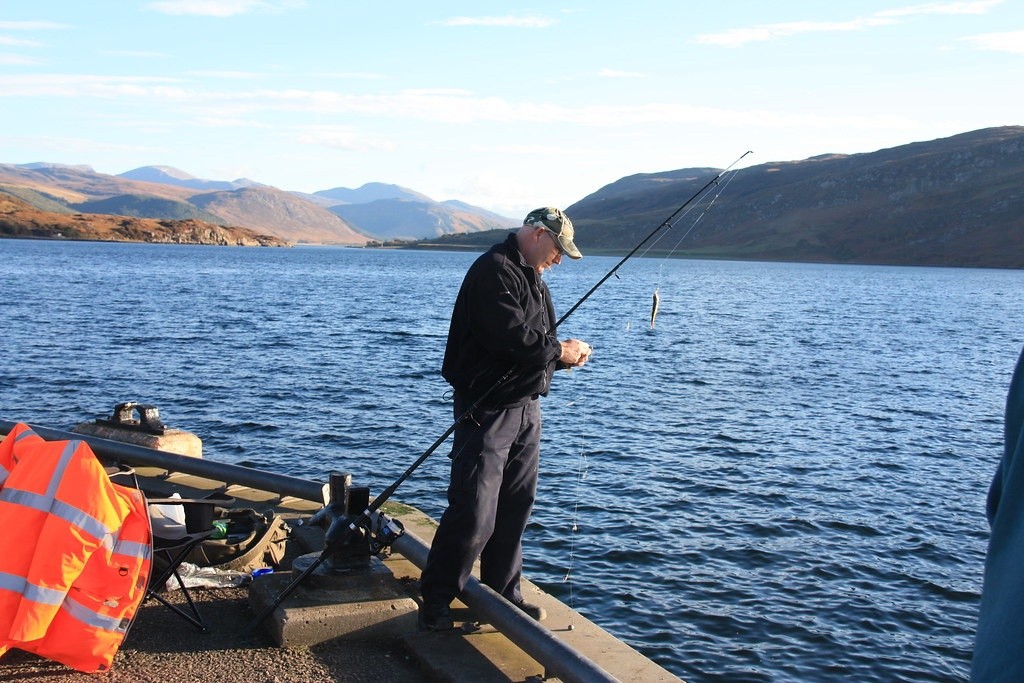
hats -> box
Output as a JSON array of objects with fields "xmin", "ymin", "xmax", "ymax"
[{"xmin": 524, "ymin": 207, "xmax": 583, "ymax": 259}]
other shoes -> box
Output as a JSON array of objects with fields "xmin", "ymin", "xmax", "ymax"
[
  {"xmin": 420, "ymin": 597, "xmax": 454, "ymax": 631},
  {"xmin": 515, "ymin": 599, "xmax": 547, "ymax": 621}
]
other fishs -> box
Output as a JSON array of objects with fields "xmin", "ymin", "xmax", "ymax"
[{"xmin": 650, "ymin": 291, "xmax": 660, "ymax": 329}]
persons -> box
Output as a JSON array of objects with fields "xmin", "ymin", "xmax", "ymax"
[{"xmin": 417, "ymin": 207, "xmax": 593, "ymax": 632}]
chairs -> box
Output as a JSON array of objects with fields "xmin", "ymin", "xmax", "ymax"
[{"xmin": 12, "ymin": 422, "xmax": 215, "ymax": 635}]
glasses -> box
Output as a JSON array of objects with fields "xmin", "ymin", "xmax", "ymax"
[{"xmin": 534, "ymin": 228, "xmax": 564, "ymax": 256}]
